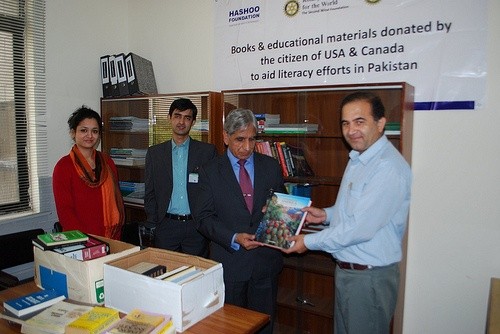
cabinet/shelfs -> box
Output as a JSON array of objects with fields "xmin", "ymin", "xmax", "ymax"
[{"xmin": 100, "ymin": 82, "xmax": 415, "ymax": 334}]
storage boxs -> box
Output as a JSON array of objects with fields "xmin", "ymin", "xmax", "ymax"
[
  {"xmin": 102, "ymin": 247, "xmax": 225, "ymax": 333},
  {"xmin": 33, "ymin": 234, "xmax": 141, "ymax": 307}
]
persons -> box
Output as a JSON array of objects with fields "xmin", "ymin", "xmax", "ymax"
[
  {"xmin": 281, "ymin": 92, "xmax": 412, "ymax": 334},
  {"xmin": 52, "ymin": 104, "xmax": 125, "ymax": 240},
  {"xmin": 194, "ymin": 108, "xmax": 289, "ymax": 334},
  {"xmin": 143, "ymin": 98, "xmax": 219, "ymax": 260}
]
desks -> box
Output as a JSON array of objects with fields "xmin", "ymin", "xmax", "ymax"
[{"xmin": 0, "ymin": 281, "xmax": 272, "ymax": 334}]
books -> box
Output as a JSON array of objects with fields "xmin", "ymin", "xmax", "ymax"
[
  {"xmin": 53, "ymin": 235, "xmax": 110, "ymax": 261},
  {"xmin": 119, "ymin": 179, "xmax": 144, "ymax": 203},
  {"xmin": 108, "ymin": 117, "xmax": 149, "ymax": 132},
  {"xmin": 253, "ymin": 191, "xmax": 312, "ymax": 249},
  {"xmin": 254, "ymin": 114, "xmax": 318, "ymax": 133},
  {"xmin": 284, "ymin": 182, "xmax": 312, "ymax": 199},
  {"xmin": 127, "ymin": 261, "xmax": 204, "ymax": 285},
  {"xmin": 255, "ymin": 140, "xmax": 316, "ymax": 177},
  {"xmin": 3, "ymin": 291, "xmax": 66, "ymax": 317},
  {"xmin": 32, "ymin": 230, "xmax": 89, "ymax": 251},
  {"xmin": 21, "ymin": 301, "xmax": 176, "ymax": 334},
  {"xmin": 384, "ymin": 122, "xmax": 400, "ymax": 135},
  {"xmin": 110, "ymin": 148, "xmax": 147, "ymax": 165}
]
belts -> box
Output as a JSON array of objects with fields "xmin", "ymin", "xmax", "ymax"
[
  {"xmin": 336, "ymin": 260, "xmax": 367, "ymax": 270},
  {"xmin": 165, "ymin": 212, "xmax": 193, "ymax": 222}
]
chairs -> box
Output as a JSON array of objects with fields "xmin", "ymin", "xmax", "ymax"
[{"xmin": 0, "ymin": 228, "xmax": 48, "ymax": 292}]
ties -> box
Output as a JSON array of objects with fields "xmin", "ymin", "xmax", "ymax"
[{"xmin": 237, "ymin": 159, "xmax": 253, "ymax": 215}]
